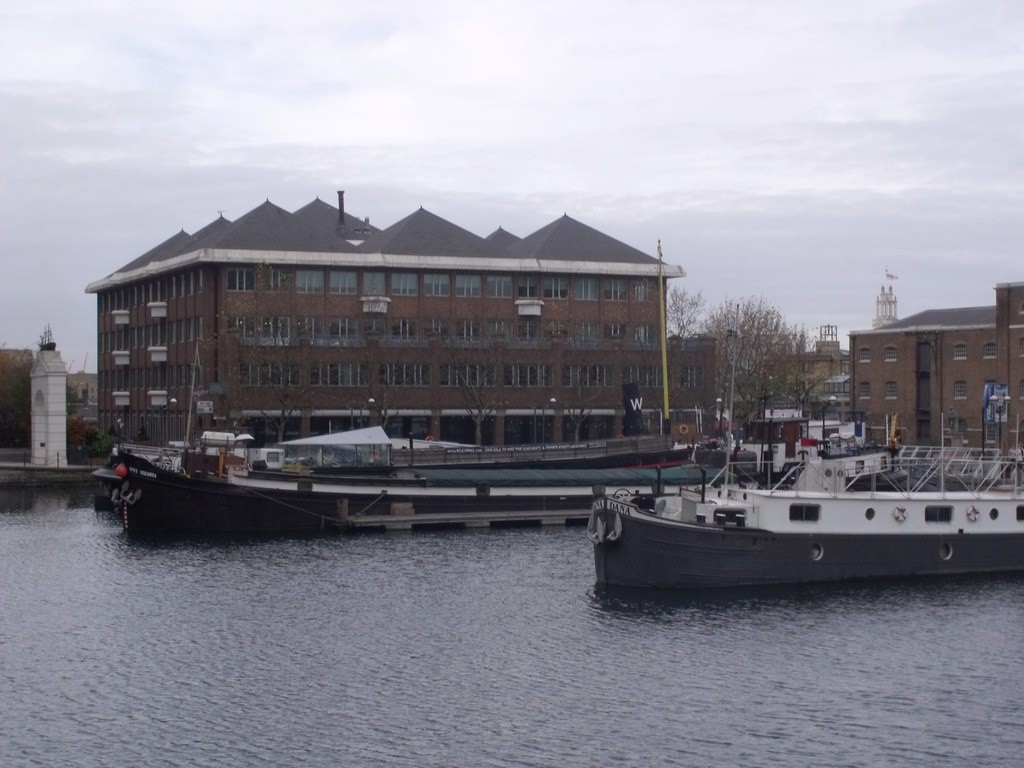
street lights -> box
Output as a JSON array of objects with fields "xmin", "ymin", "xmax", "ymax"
[
  {"xmin": 359, "ymin": 398, "xmax": 376, "ymax": 427},
  {"xmin": 541, "ymin": 397, "xmax": 557, "ymax": 444},
  {"xmin": 988, "ymin": 393, "xmax": 1011, "ymax": 483}
]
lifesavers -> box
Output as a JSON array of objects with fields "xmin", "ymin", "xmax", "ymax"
[
  {"xmin": 426, "ymin": 436, "xmax": 434, "ymax": 441},
  {"xmin": 967, "ymin": 505, "xmax": 980, "ymax": 521},
  {"xmin": 894, "ymin": 505, "xmax": 906, "ymax": 521},
  {"xmin": 679, "ymin": 424, "xmax": 688, "ymax": 435}
]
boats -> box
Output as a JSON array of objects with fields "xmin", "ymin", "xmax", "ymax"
[
  {"xmin": 91, "ymin": 337, "xmax": 742, "ymax": 532},
  {"xmin": 586, "ymin": 303, "xmax": 1024, "ymax": 592}
]
